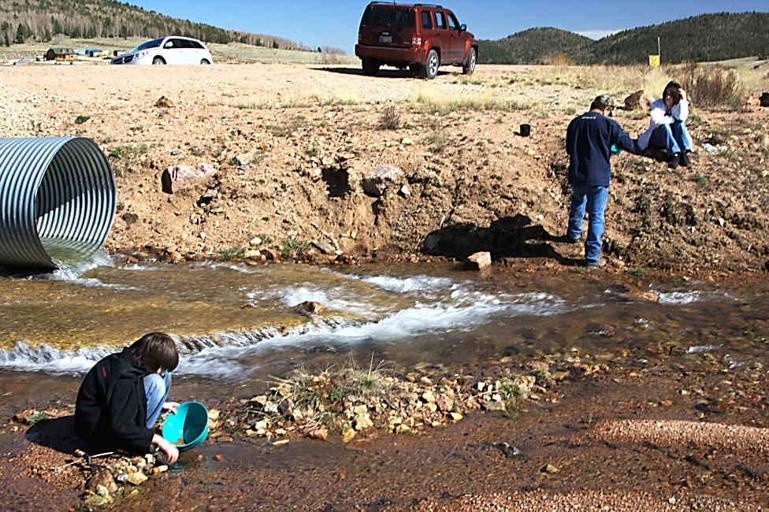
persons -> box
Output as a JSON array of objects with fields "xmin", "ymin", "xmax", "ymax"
[
  {"xmin": 638, "ymin": 81, "xmax": 694, "ymax": 168},
  {"xmin": 566, "ymin": 96, "xmax": 637, "ymax": 270},
  {"xmin": 75, "ymin": 333, "xmax": 179, "ymax": 465}
]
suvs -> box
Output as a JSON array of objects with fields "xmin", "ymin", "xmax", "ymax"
[
  {"xmin": 111, "ymin": 36, "xmax": 213, "ymax": 66},
  {"xmin": 354, "ymin": 1, "xmax": 480, "ymax": 80}
]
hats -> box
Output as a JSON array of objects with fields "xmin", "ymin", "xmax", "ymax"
[{"xmin": 595, "ymin": 93, "xmax": 615, "ymax": 108}]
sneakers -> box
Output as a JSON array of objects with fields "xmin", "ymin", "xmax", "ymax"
[
  {"xmin": 681, "ymin": 153, "xmax": 690, "ymax": 166},
  {"xmin": 669, "ymin": 155, "xmax": 681, "ymax": 168}
]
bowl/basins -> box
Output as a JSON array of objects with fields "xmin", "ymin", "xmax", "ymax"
[
  {"xmin": 611, "ymin": 145, "xmax": 624, "ymax": 154},
  {"xmin": 162, "ymin": 400, "xmax": 208, "ymax": 450}
]
